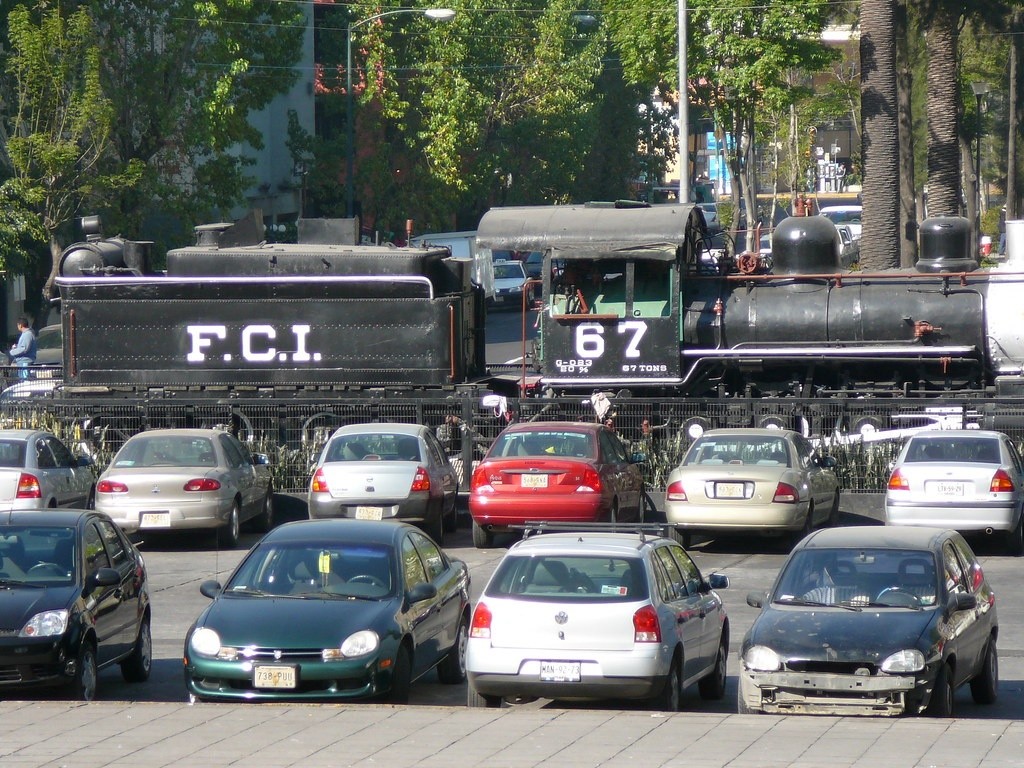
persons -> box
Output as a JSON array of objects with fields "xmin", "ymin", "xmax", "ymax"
[{"xmin": 10, "ymin": 316, "xmax": 37, "ymax": 381}]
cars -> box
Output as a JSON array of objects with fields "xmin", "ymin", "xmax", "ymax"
[
  {"xmin": 691, "ymin": 206, "xmax": 863, "ymax": 274},
  {"xmin": 180, "ymin": 519, "xmax": 470, "ymax": 705},
  {"xmin": 0, "ymin": 429, "xmax": 98, "ymax": 555},
  {"xmin": 0, "ymin": 510, "xmax": 153, "ymax": 703},
  {"xmin": 465, "ymin": 529, "xmax": 732, "ymax": 712},
  {"xmin": 306, "ymin": 423, "xmax": 458, "ymax": 543},
  {"xmin": 883, "ymin": 428, "xmax": 1023, "ymax": 552},
  {"xmin": 666, "ymin": 427, "xmax": 841, "ymax": 552},
  {"xmin": 412, "ymin": 230, "xmax": 566, "ymax": 309},
  {"xmin": 1, "ymin": 324, "xmax": 63, "ymax": 419},
  {"xmin": 739, "ymin": 524, "xmax": 1000, "ymax": 716},
  {"xmin": 93, "ymin": 428, "xmax": 276, "ymax": 547},
  {"xmin": 468, "ymin": 421, "xmax": 648, "ymax": 549}
]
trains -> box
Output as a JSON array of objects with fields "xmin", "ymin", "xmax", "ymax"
[{"xmin": 40, "ymin": 200, "xmax": 1023, "ymax": 444}]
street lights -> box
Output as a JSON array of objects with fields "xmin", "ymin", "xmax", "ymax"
[
  {"xmin": 969, "ymin": 78, "xmax": 991, "ymax": 262},
  {"xmin": 343, "ymin": 9, "xmax": 456, "ymax": 221}
]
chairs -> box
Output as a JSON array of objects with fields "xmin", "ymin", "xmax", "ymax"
[
  {"xmin": 0, "ymin": 536, "xmax": 31, "ymax": 580},
  {"xmin": 803, "ymin": 561, "xmax": 875, "ymax": 603},
  {"xmin": 526, "ymin": 559, "xmax": 575, "ymax": 593},
  {"xmin": 343, "ymin": 442, "xmax": 366, "ymax": 460},
  {"xmin": 398, "ymin": 438, "xmax": 418, "ymax": 459},
  {"xmin": 517, "ymin": 441, "xmax": 537, "ymax": 456},
  {"xmin": 281, "ymin": 555, "xmax": 319, "ymax": 594},
  {"xmin": 895, "ymin": 559, "xmax": 936, "ymax": 605}
]
